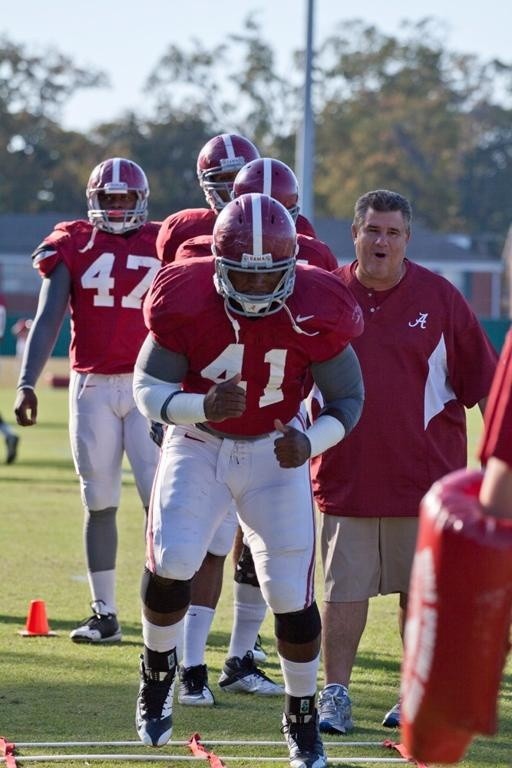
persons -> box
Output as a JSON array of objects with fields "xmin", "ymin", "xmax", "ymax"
[
  {"xmin": 397, "ymin": 325, "xmax": 510, "ymax": 762},
  {"xmin": 307, "ymin": 191, "xmax": 501, "ymax": 734},
  {"xmin": 0, "ymin": 294, "xmax": 18, "ymax": 464},
  {"xmin": 153, "ymin": 133, "xmax": 316, "ymax": 268},
  {"xmin": 131, "ymin": 192, "xmax": 365, "ymax": 768},
  {"xmin": 12, "ymin": 157, "xmax": 162, "ymax": 644},
  {"xmin": 175, "ymin": 158, "xmax": 339, "ymax": 707}
]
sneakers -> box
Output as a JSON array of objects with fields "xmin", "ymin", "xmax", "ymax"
[
  {"xmin": 382, "ymin": 704, "xmax": 400, "ymax": 728},
  {"xmin": 281, "ymin": 710, "xmax": 326, "ymax": 767},
  {"xmin": 316, "ymin": 681, "xmax": 355, "ymax": 733},
  {"xmin": 72, "ymin": 614, "xmax": 123, "ymax": 644},
  {"xmin": 252, "ymin": 636, "xmax": 267, "ymax": 664},
  {"xmin": 134, "ymin": 651, "xmax": 180, "ymax": 749},
  {"xmin": 218, "ymin": 654, "xmax": 285, "ymax": 694},
  {"xmin": 178, "ymin": 665, "xmax": 216, "ymax": 706}
]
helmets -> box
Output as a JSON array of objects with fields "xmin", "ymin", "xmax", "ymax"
[
  {"xmin": 197, "ymin": 133, "xmax": 260, "ymax": 215},
  {"xmin": 210, "ymin": 192, "xmax": 298, "ymax": 316},
  {"xmin": 86, "ymin": 158, "xmax": 150, "ymax": 235},
  {"xmin": 229, "ymin": 156, "xmax": 300, "ymax": 225}
]
